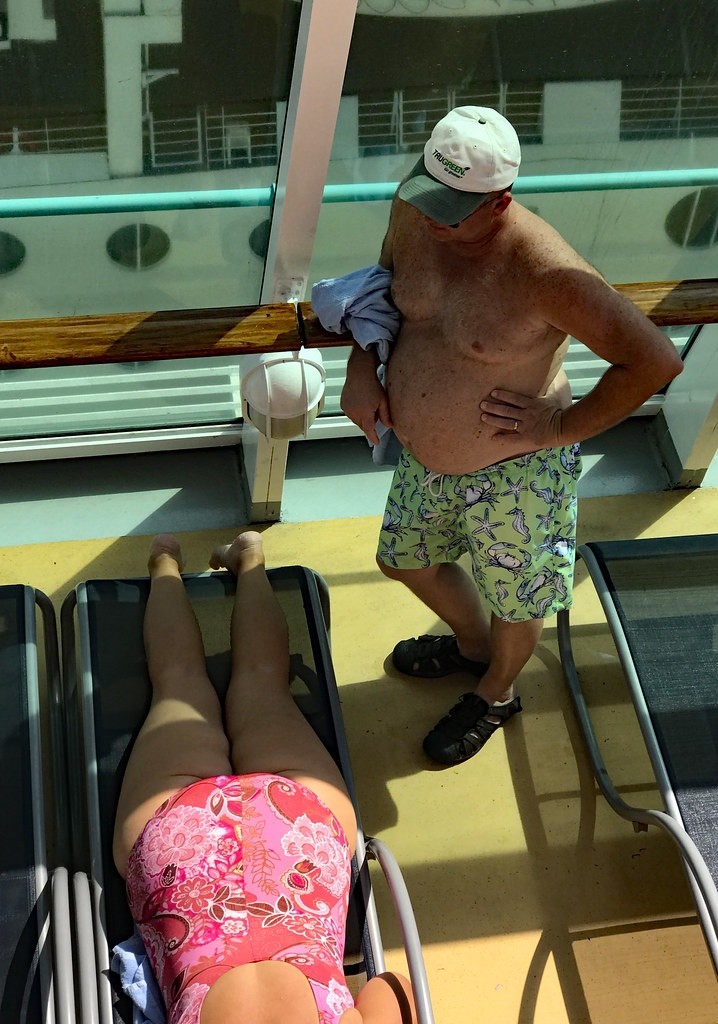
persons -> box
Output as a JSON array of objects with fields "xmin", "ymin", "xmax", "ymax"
[
  {"xmin": 111, "ymin": 532, "xmax": 418, "ymax": 1024},
  {"xmin": 340, "ymin": 105, "xmax": 683, "ymax": 766}
]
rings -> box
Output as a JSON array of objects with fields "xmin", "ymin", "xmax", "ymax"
[{"xmin": 514, "ymin": 422, "xmax": 518, "ymax": 430}]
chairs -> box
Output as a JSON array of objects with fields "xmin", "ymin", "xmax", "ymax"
[
  {"xmin": 0, "ymin": 567, "xmax": 434, "ymax": 1024},
  {"xmin": 556, "ymin": 532, "xmax": 718, "ymax": 976}
]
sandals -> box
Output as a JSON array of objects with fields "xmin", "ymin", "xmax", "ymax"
[
  {"xmin": 422, "ymin": 691, "xmax": 522, "ymax": 767},
  {"xmin": 392, "ymin": 633, "xmax": 490, "ymax": 679}
]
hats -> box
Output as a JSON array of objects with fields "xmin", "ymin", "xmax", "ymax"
[{"xmin": 396, "ymin": 106, "xmax": 521, "ymax": 226}]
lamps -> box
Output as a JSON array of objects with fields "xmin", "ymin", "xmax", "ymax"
[{"xmin": 240, "ymin": 347, "xmax": 326, "ymax": 446}]
